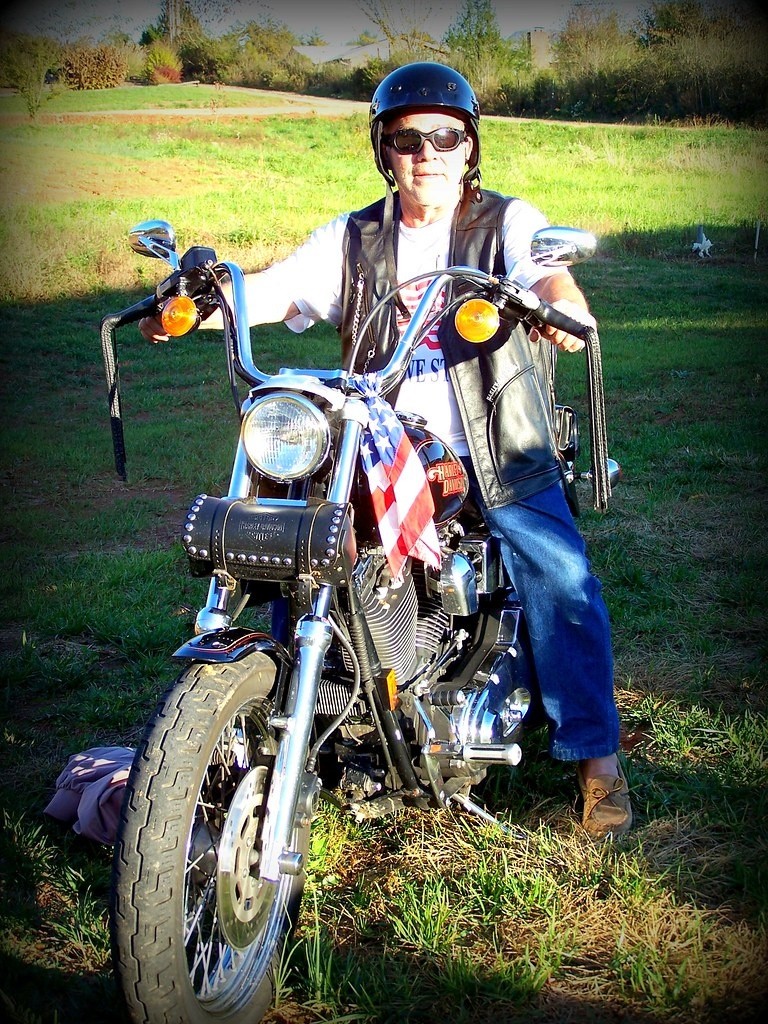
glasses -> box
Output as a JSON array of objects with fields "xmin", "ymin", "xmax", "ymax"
[{"xmin": 379, "ymin": 126, "xmax": 467, "ymax": 155}]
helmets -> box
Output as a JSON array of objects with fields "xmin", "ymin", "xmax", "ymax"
[{"xmin": 369, "ymin": 62, "xmax": 484, "ymax": 188}]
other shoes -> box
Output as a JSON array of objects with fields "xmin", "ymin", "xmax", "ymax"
[{"xmin": 574, "ymin": 755, "xmax": 632, "ymax": 838}]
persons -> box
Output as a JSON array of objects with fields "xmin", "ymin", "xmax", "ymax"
[{"xmin": 137, "ymin": 61, "xmax": 633, "ymax": 830}]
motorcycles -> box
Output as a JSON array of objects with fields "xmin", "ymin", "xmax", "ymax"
[{"xmin": 96, "ymin": 212, "xmax": 620, "ymax": 1024}]
